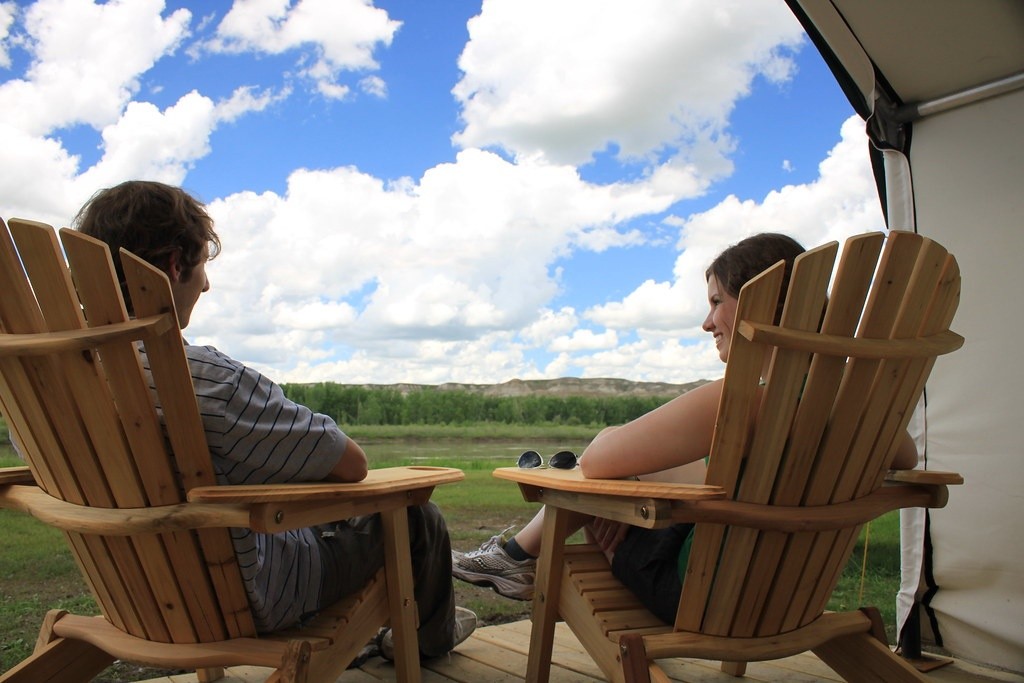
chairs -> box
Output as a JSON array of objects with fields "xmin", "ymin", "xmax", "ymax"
[
  {"xmin": 0, "ymin": 217, "xmax": 465, "ymax": 683},
  {"xmin": 491, "ymin": 232, "xmax": 965, "ymax": 683}
]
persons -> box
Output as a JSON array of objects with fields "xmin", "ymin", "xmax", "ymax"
[
  {"xmin": 450, "ymin": 232, "xmax": 920, "ymax": 627},
  {"xmin": 9, "ymin": 180, "xmax": 477, "ymax": 664}
]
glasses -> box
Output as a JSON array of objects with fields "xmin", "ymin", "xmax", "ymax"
[{"xmin": 516, "ymin": 450, "xmax": 579, "ymax": 470}]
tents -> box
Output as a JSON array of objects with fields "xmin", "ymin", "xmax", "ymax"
[{"xmin": 783, "ymin": 0, "xmax": 1024, "ymax": 683}]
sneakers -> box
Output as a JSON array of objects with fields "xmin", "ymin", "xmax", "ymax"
[
  {"xmin": 346, "ymin": 627, "xmax": 387, "ymax": 670},
  {"xmin": 452, "ymin": 525, "xmax": 537, "ymax": 601},
  {"xmin": 376, "ymin": 606, "xmax": 477, "ymax": 664}
]
